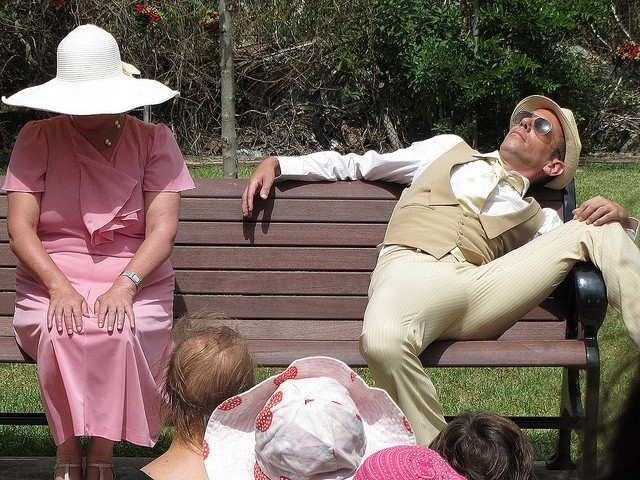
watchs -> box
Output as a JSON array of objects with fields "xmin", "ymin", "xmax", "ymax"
[{"xmin": 120, "ymin": 272, "xmax": 143, "ymax": 290}]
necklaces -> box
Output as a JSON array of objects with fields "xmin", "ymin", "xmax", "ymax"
[{"xmin": 63, "ymin": 115, "xmax": 122, "ymax": 153}]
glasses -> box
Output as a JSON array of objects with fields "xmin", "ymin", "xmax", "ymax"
[{"xmin": 512, "ymin": 110, "xmax": 561, "ymax": 160}]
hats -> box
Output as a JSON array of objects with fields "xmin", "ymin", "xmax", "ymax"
[
  {"xmin": 510, "ymin": 95, "xmax": 582, "ymax": 189},
  {"xmin": 1, "ymin": 24, "xmax": 179, "ymax": 116}
]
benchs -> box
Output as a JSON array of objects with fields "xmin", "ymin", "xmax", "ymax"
[{"xmin": 0, "ymin": 172, "xmax": 607, "ymax": 480}]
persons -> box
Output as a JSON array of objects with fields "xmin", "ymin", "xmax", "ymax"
[
  {"xmin": 429, "ymin": 410, "xmax": 535, "ymax": 479},
  {"xmin": 240, "ymin": 93, "xmax": 639, "ymax": 447},
  {"xmin": 1, "ymin": 23, "xmax": 195, "ymax": 479},
  {"xmin": 114, "ymin": 303, "xmax": 256, "ymax": 479}
]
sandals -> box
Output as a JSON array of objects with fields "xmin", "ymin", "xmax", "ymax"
[
  {"xmin": 83, "ymin": 461, "xmax": 117, "ymax": 479},
  {"xmin": 53, "ymin": 461, "xmax": 83, "ymax": 480}
]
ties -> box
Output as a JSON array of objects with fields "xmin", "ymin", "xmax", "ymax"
[{"xmin": 455, "ymin": 160, "xmax": 523, "ymax": 216}]
